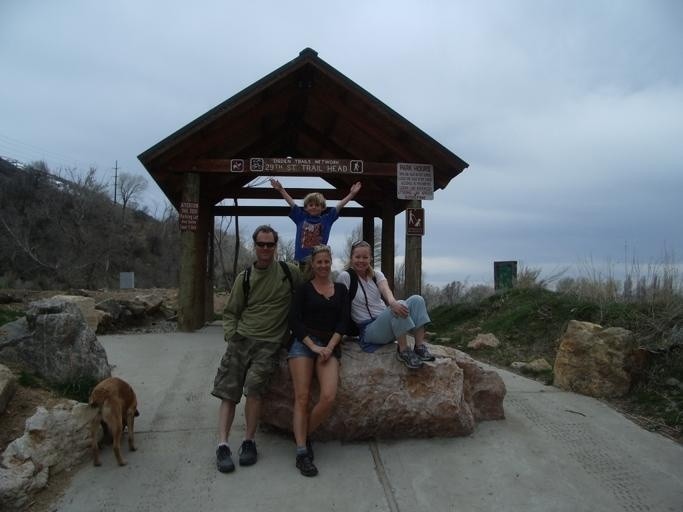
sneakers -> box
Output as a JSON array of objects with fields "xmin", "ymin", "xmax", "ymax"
[
  {"xmin": 396, "ymin": 344, "xmax": 435, "ymax": 368},
  {"xmin": 239, "ymin": 440, "xmax": 257, "ymax": 466},
  {"xmin": 216, "ymin": 445, "xmax": 235, "ymax": 472},
  {"xmin": 296, "ymin": 435, "xmax": 317, "ymax": 476}
]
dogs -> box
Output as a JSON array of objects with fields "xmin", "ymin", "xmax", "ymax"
[{"xmin": 81, "ymin": 375, "xmax": 141, "ymax": 467}]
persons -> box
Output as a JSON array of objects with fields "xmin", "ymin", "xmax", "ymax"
[
  {"xmin": 211, "ymin": 225, "xmax": 304, "ymax": 474},
  {"xmin": 336, "ymin": 241, "xmax": 436, "ymax": 369},
  {"xmin": 288, "ymin": 244, "xmax": 350, "ymax": 477},
  {"xmin": 268, "ymin": 177, "xmax": 361, "ymax": 284}
]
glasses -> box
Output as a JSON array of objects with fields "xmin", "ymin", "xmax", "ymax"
[
  {"xmin": 352, "ymin": 240, "xmax": 371, "ymax": 248},
  {"xmin": 256, "ymin": 242, "xmax": 276, "ymax": 247}
]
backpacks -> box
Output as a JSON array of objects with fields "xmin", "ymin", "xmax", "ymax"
[{"xmin": 340, "ymin": 267, "xmax": 384, "ymax": 337}]
